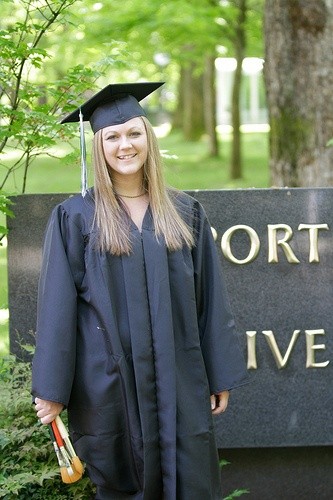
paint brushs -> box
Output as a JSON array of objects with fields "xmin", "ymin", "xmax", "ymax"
[{"xmin": 33, "ymin": 398, "xmax": 86, "ymax": 485}]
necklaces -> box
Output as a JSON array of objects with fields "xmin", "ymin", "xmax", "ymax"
[{"xmin": 114, "ymin": 191, "xmax": 148, "ymax": 198}]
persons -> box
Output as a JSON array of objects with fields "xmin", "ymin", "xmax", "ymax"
[{"xmin": 29, "ymin": 81, "xmax": 249, "ymax": 500}]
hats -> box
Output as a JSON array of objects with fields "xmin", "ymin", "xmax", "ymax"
[{"xmin": 60, "ymin": 82, "xmax": 166, "ymax": 198}]
